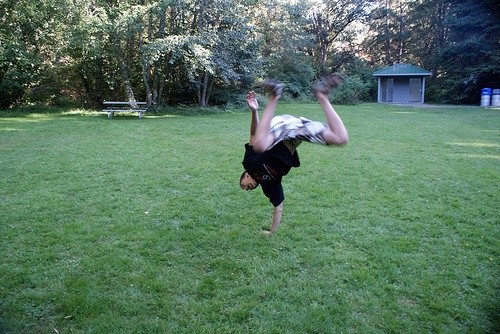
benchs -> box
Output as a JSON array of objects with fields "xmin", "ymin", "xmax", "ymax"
[{"xmin": 102, "ymin": 109, "xmax": 148, "ymax": 119}]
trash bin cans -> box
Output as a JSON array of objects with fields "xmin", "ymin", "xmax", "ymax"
[
  {"xmin": 481, "ymin": 88, "xmax": 492, "ymax": 107},
  {"xmin": 491, "ymin": 88, "xmax": 500, "ymax": 106}
]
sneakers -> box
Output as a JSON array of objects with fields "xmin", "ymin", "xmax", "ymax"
[
  {"xmin": 258, "ymin": 80, "xmax": 286, "ymax": 97},
  {"xmin": 310, "ymin": 72, "xmax": 344, "ymax": 95}
]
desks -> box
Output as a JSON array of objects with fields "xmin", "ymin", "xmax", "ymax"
[{"xmin": 102, "ymin": 101, "xmax": 148, "ymax": 120}]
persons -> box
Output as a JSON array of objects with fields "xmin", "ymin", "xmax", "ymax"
[{"xmin": 239, "ymin": 73, "xmax": 350, "ymax": 236}]
